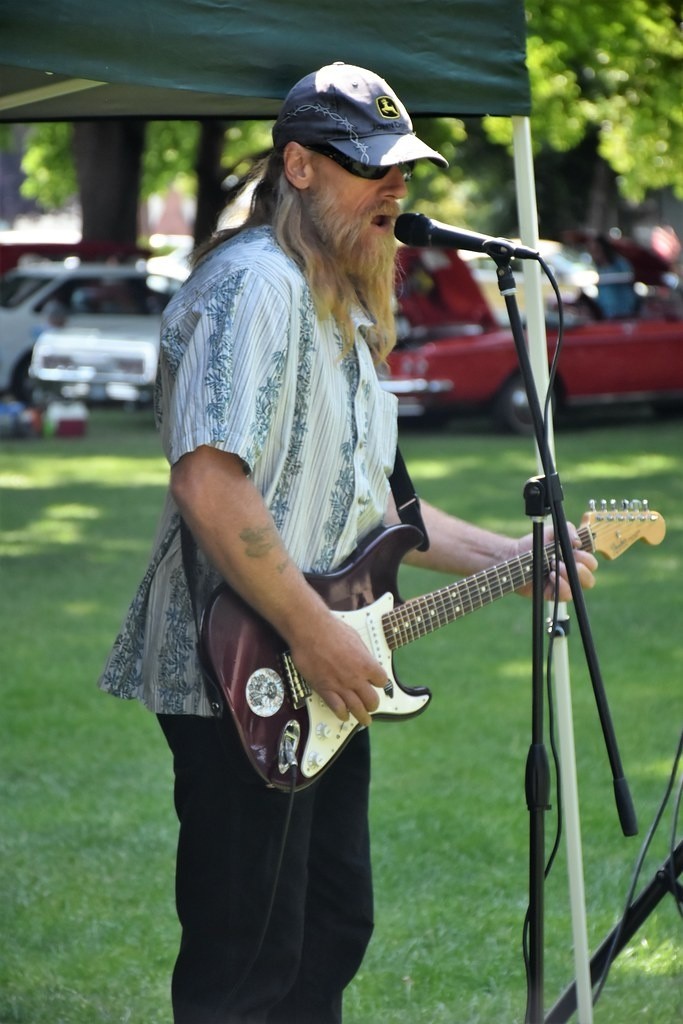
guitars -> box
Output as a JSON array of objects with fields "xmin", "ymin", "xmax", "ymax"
[{"xmin": 201, "ymin": 497, "xmax": 667, "ymax": 795}]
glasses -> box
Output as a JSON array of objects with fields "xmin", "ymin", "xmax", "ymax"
[{"xmin": 305, "ymin": 146, "xmax": 415, "ymax": 181}]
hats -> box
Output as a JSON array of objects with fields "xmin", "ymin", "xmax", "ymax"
[{"xmin": 272, "ymin": 60, "xmax": 449, "ymax": 170}]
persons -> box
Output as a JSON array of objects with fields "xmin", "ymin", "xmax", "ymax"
[{"xmin": 94, "ymin": 61, "xmax": 597, "ymax": 1024}]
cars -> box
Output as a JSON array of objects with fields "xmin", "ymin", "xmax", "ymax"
[
  {"xmin": 0, "ymin": 264, "xmax": 190, "ymax": 408},
  {"xmin": 382, "ymin": 318, "xmax": 682, "ymax": 437}
]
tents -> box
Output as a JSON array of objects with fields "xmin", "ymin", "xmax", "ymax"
[{"xmin": 0, "ymin": 0, "xmax": 594, "ymax": 1024}]
